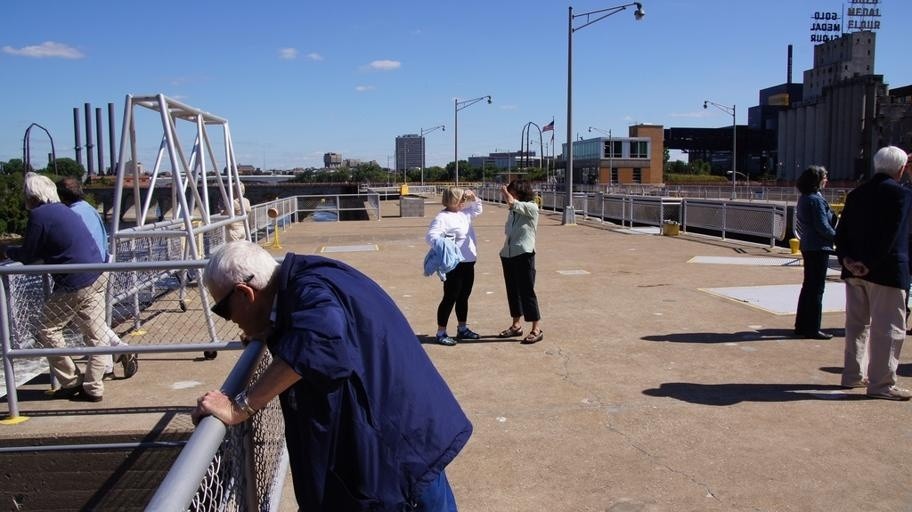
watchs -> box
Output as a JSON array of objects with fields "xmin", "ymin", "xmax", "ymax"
[{"xmin": 232, "ymin": 386, "xmax": 255, "ymax": 419}]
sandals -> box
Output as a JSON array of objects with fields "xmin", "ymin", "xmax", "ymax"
[
  {"xmin": 524, "ymin": 330, "xmax": 543, "ymax": 343},
  {"xmin": 500, "ymin": 326, "xmax": 523, "ymax": 338}
]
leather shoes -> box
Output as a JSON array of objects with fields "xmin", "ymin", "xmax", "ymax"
[
  {"xmin": 69, "ymin": 386, "xmax": 102, "ymax": 402},
  {"xmin": 793, "ymin": 328, "xmax": 805, "ymax": 338},
  {"xmin": 805, "ymin": 330, "xmax": 833, "ymax": 339},
  {"xmin": 43, "ymin": 381, "xmax": 83, "ymax": 400}
]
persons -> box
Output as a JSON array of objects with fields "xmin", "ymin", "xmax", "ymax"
[
  {"xmin": 424, "ymin": 185, "xmax": 483, "ymax": 346},
  {"xmin": 0, "ymin": 171, "xmax": 105, "ymax": 401},
  {"xmin": 192, "ymin": 241, "xmax": 473, "ymax": 512},
  {"xmin": 56, "ymin": 177, "xmax": 139, "ymax": 380},
  {"xmin": 795, "ymin": 165, "xmax": 837, "ymax": 340},
  {"xmin": 221, "ymin": 180, "xmax": 251, "ymax": 241},
  {"xmin": 836, "ymin": 147, "xmax": 911, "ymax": 401},
  {"xmin": 499, "ymin": 179, "xmax": 543, "ymax": 344}
]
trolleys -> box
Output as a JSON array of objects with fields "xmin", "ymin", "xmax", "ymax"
[{"xmin": 99, "ymin": 94, "xmax": 254, "ymax": 363}]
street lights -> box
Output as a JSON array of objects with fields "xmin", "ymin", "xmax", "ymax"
[
  {"xmin": 562, "ymin": 2, "xmax": 645, "ymax": 226},
  {"xmin": 530, "ymin": 140, "xmax": 550, "ymax": 184},
  {"xmin": 703, "ymin": 100, "xmax": 736, "ymax": 199},
  {"xmin": 455, "ymin": 95, "xmax": 492, "ymax": 186},
  {"xmin": 421, "ymin": 125, "xmax": 445, "ymax": 186},
  {"xmin": 588, "ymin": 126, "xmax": 612, "ymax": 187}
]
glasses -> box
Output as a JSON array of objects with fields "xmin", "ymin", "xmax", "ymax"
[{"xmin": 211, "ymin": 274, "xmax": 255, "ymax": 320}]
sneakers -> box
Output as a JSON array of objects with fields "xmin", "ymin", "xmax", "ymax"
[
  {"xmin": 457, "ymin": 328, "xmax": 479, "ymax": 339},
  {"xmin": 841, "ymin": 378, "xmax": 869, "ymax": 388},
  {"xmin": 867, "ymin": 386, "xmax": 912, "ymax": 400},
  {"xmin": 436, "ymin": 333, "xmax": 457, "ymax": 345},
  {"xmin": 80, "ymin": 371, "xmax": 114, "ymax": 381},
  {"xmin": 122, "ymin": 352, "xmax": 138, "ymax": 378}
]
